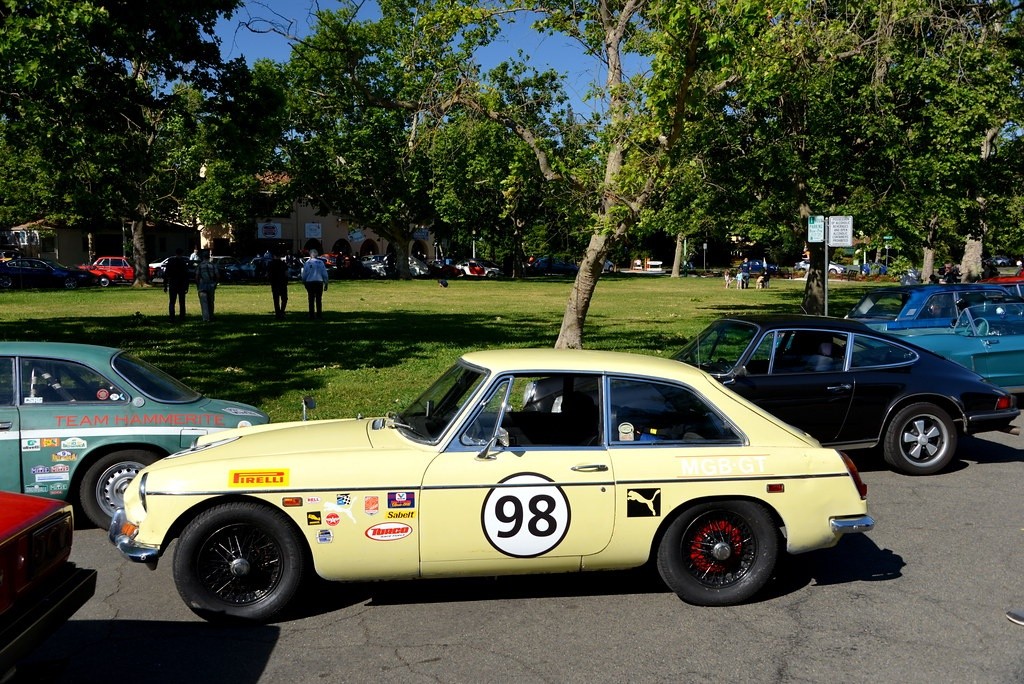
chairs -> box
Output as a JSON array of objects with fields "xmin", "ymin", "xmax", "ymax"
[
  {"xmin": 556, "ymin": 392, "xmax": 596, "ymax": 445},
  {"xmin": 786, "ymin": 333, "xmax": 841, "ymax": 371}
]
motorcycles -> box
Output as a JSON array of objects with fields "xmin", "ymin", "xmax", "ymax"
[{"xmin": 900, "ymin": 266, "xmax": 922, "ymax": 286}]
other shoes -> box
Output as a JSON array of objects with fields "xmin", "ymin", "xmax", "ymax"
[
  {"xmin": 168, "ymin": 313, "xmax": 186, "ymax": 318},
  {"xmin": 276, "ymin": 311, "xmax": 286, "ymax": 317},
  {"xmin": 202, "ymin": 313, "xmax": 214, "ymax": 323},
  {"xmin": 310, "ymin": 310, "xmax": 322, "ymax": 315}
]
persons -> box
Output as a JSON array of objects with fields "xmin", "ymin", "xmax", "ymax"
[
  {"xmin": 163, "ymin": 247, "xmax": 190, "ymax": 325},
  {"xmin": 938, "ymin": 260, "xmax": 957, "ymax": 284},
  {"xmin": 725, "ymin": 258, "xmax": 770, "ymax": 290},
  {"xmin": 196, "ymin": 251, "xmax": 220, "ymax": 322},
  {"xmin": 189, "ymin": 249, "xmax": 200, "ymax": 261},
  {"xmin": 266, "ymin": 251, "xmax": 288, "ymax": 319},
  {"xmin": 302, "ymin": 249, "xmax": 329, "ymax": 320}
]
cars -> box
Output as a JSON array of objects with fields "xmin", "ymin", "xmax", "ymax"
[
  {"xmin": 0, "ymin": 489, "xmax": 98, "ymax": 684},
  {"xmin": 0, "ymin": 341, "xmax": 271, "ymax": 532},
  {"xmin": 209, "ymin": 256, "xmax": 339, "ymax": 281},
  {"xmin": 428, "ymin": 260, "xmax": 444, "ymax": 269},
  {"xmin": 79, "ymin": 257, "xmax": 156, "ymax": 288},
  {"xmin": 110, "ymin": 349, "xmax": 874, "ymax": 623},
  {"xmin": 889, "ymin": 302, "xmax": 1024, "ymax": 386},
  {"xmin": 454, "ymin": 261, "xmax": 499, "ymax": 277},
  {"xmin": 604, "ymin": 260, "xmax": 614, "ymax": 271},
  {"xmin": 148, "ymin": 256, "xmax": 197, "ymax": 279},
  {"xmin": 740, "ymin": 260, "xmax": 778, "ymax": 271},
  {"xmin": 844, "ymin": 277, "xmax": 1024, "ymax": 334},
  {"xmin": 526, "ymin": 313, "xmax": 1020, "ymax": 476},
  {"xmin": 360, "ymin": 255, "xmax": 384, "ymax": 264},
  {"xmin": 860, "ymin": 263, "xmax": 887, "ymax": 274},
  {"xmin": 794, "ymin": 259, "xmax": 847, "ymax": 275},
  {"xmin": 531, "ymin": 258, "xmax": 578, "ymax": 273},
  {"xmin": 0, "ymin": 258, "xmax": 88, "ymax": 290},
  {"xmin": 984, "ymin": 257, "xmax": 1010, "ymax": 266}
]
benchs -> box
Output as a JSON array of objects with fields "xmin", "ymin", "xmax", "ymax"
[{"xmin": 841, "ymin": 269, "xmax": 859, "ymax": 282}]
